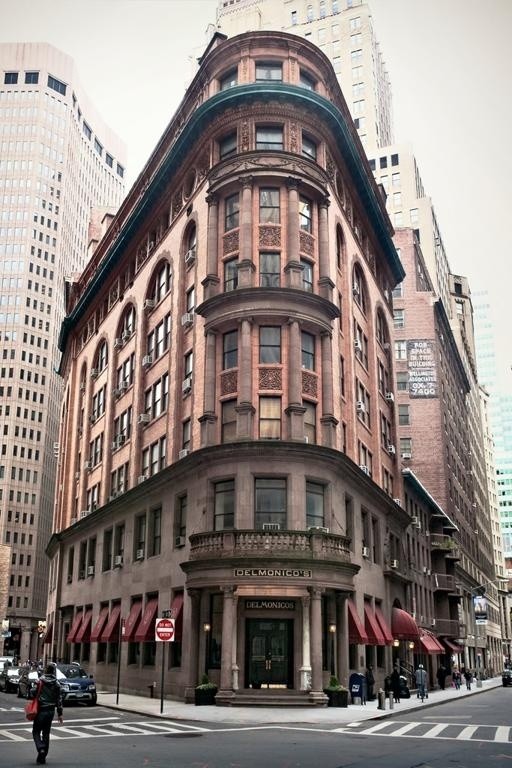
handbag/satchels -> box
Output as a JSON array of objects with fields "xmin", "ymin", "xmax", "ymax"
[{"xmin": 25, "ymin": 699, "xmax": 38, "ymax": 720}]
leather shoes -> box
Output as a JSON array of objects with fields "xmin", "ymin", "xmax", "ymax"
[{"xmin": 37, "ymin": 750, "xmax": 45, "ymax": 764}]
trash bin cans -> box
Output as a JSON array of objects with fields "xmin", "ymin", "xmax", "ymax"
[
  {"xmin": 349, "ymin": 673, "xmax": 368, "ymax": 705},
  {"xmin": 377, "ymin": 691, "xmax": 386, "ymax": 710}
]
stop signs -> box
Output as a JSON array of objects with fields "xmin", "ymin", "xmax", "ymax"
[{"xmin": 155, "ymin": 618, "xmax": 175, "ymax": 642}]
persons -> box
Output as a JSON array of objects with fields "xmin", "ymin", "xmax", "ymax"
[
  {"xmin": 464, "ymin": 669, "xmax": 472, "ymax": 690},
  {"xmin": 31, "ymin": 664, "xmax": 63, "ymax": 764},
  {"xmin": 452, "ymin": 667, "xmax": 461, "ymax": 690},
  {"xmin": 390, "ymin": 668, "xmax": 401, "ymax": 704},
  {"xmin": 366, "ymin": 664, "xmax": 376, "ymax": 702},
  {"xmin": 436, "ymin": 664, "xmax": 448, "ymax": 690},
  {"xmin": 415, "ymin": 664, "xmax": 427, "ymax": 700}
]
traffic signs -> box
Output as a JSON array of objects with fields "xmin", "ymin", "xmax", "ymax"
[{"xmin": 162, "ymin": 609, "xmax": 172, "ymax": 618}]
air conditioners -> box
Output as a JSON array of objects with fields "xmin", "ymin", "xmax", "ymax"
[
  {"xmin": 362, "ymin": 466, "xmax": 368, "ymax": 474},
  {"xmin": 387, "ymin": 393, "xmax": 395, "ymax": 403},
  {"xmin": 388, "ymin": 445, "xmax": 395, "ymax": 453},
  {"xmin": 114, "ymin": 338, "xmax": 123, "ymax": 348},
  {"xmin": 175, "ymin": 535, "xmax": 185, "ymax": 546},
  {"xmin": 119, "ymin": 382, "xmax": 128, "ymax": 391},
  {"xmin": 113, "ymin": 389, "xmax": 121, "ymax": 397},
  {"xmin": 51, "ymin": 408, "xmax": 126, "ymax": 546},
  {"xmin": 182, "ymin": 313, "xmax": 192, "ymax": 327},
  {"xmin": 354, "ymin": 340, "xmax": 362, "ymax": 351},
  {"xmin": 136, "ymin": 549, "xmax": 143, "ymax": 557},
  {"xmin": 182, "ymin": 378, "xmax": 191, "ymax": 393},
  {"xmin": 361, "ymin": 546, "xmax": 369, "ymax": 558},
  {"xmin": 122, "ymin": 330, "xmax": 130, "ymax": 340},
  {"xmin": 358, "ymin": 402, "xmax": 365, "ymax": 413},
  {"xmin": 80, "ymin": 382, "xmax": 86, "ymax": 390},
  {"xmin": 91, "ymin": 369, "xmax": 98, "ymax": 377},
  {"xmin": 352, "ymin": 282, "xmax": 359, "ymax": 295},
  {"xmin": 144, "ymin": 299, "xmax": 155, "ymax": 314},
  {"xmin": 395, "ymin": 499, "xmax": 400, "ymax": 506},
  {"xmin": 142, "ymin": 355, "xmax": 153, "ymax": 367},
  {"xmin": 88, "ymin": 566, "xmax": 94, "ymax": 573},
  {"xmin": 115, "ymin": 555, "xmax": 124, "ymax": 568},
  {"xmin": 179, "ymin": 449, "xmax": 189, "ymax": 459},
  {"xmin": 137, "ymin": 414, "xmax": 150, "ymax": 425},
  {"xmin": 138, "ymin": 476, "xmax": 146, "ymax": 484},
  {"xmin": 400, "ymin": 452, "xmax": 488, "ymax": 631},
  {"xmin": 383, "ymin": 343, "xmax": 390, "ymax": 352},
  {"xmin": 184, "ymin": 250, "xmax": 195, "ymax": 264},
  {"xmin": 391, "ymin": 560, "xmax": 400, "ymax": 568}
]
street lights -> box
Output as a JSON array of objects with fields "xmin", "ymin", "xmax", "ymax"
[{"xmin": 470, "ymin": 579, "xmax": 511, "ymax": 688}]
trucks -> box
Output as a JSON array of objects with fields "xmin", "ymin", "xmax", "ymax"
[{"xmin": 502, "ymin": 659, "xmax": 512, "ymax": 687}]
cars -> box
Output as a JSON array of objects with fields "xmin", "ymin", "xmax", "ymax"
[{"xmin": 0, "ymin": 654, "xmax": 43, "ymax": 700}]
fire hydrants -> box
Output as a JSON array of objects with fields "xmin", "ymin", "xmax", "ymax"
[{"xmin": 376, "ymin": 686, "xmax": 386, "ymax": 709}]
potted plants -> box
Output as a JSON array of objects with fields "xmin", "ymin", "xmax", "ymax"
[
  {"xmin": 193, "ymin": 677, "xmax": 221, "ymax": 708},
  {"xmin": 324, "ymin": 672, "xmax": 351, "ymax": 709}
]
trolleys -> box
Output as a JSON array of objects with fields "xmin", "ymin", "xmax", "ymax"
[{"xmin": 417, "ymin": 680, "xmax": 429, "ymax": 698}]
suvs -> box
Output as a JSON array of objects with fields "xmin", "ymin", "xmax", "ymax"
[{"xmin": 44, "ymin": 661, "xmax": 97, "ymax": 707}]
